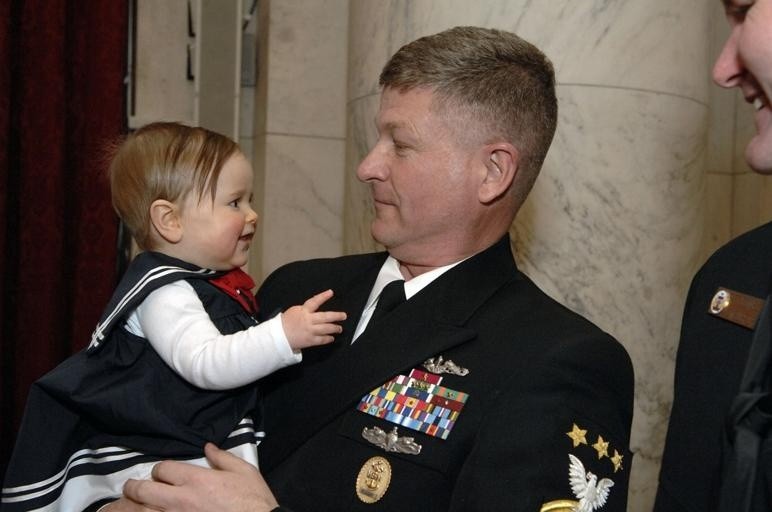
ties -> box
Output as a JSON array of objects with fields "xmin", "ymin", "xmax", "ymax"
[{"xmin": 364, "ymin": 280, "xmax": 406, "ymax": 330}]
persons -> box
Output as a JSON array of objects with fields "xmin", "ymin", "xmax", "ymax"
[
  {"xmin": 647, "ymin": 2, "xmax": 771, "ymax": 509},
  {"xmin": 1, "ymin": 119, "xmax": 350, "ymax": 512},
  {"xmin": 83, "ymin": 23, "xmax": 637, "ymax": 509}
]
